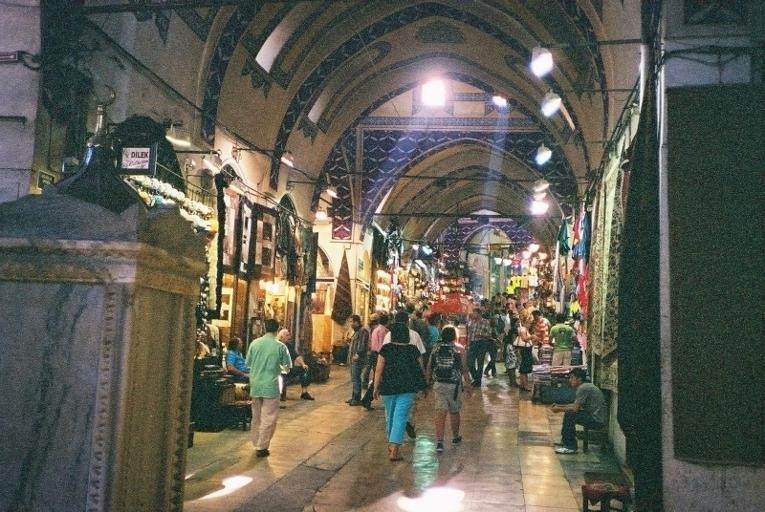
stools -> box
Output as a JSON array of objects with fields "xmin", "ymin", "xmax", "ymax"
[
  {"xmin": 579, "ymin": 465, "xmax": 634, "ymax": 512},
  {"xmin": 580, "ymin": 421, "xmax": 612, "ymax": 453}
]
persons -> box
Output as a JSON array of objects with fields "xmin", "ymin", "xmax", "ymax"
[
  {"xmin": 551, "ymin": 369, "xmax": 608, "ymax": 455},
  {"xmin": 277, "ymin": 329, "xmax": 315, "ymax": 401},
  {"xmin": 245, "ymin": 319, "xmax": 293, "ymax": 456},
  {"xmin": 345, "ymin": 293, "xmax": 574, "ymax": 461},
  {"xmin": 225, "ymin": 338, "xmax": 251, "ymax": 383}
]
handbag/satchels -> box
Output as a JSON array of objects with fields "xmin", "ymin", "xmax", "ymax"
[{"xmin": 513, "ymin": 336, "xmax": 534, "ymax": 348}]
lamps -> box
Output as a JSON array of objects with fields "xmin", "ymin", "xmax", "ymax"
[
  {"xmin": 529, "ymin": 176, "xmax": 604, "ymax": 216},
  {"xmin": 529, "ymin": 31, "xmax": 649, "ymax": 79},
  {"xmin": 315, "ymin": 205, "xmax": 329, "ymax": 220},
  {"xmin": 286, "ymin": 179, "xmax": 341, "ymax": 199},
  {"xmin": 104, "ymin": 117, "xmax": 192, "ymax": 151},
  {"xmin": 172, "ymin": 144, "xmax": 236, "ymax": 170},
  {"xmin": 536, "ymin": 86, "xmax": 643, "ymax": 119},
  {"xmin": 532, "ymin": 138, "xmax": 620, "ymax": 169},
  {"xmin": 385, "ymin": 234, "xmax": 437, "ymax": 255},
  {"xmin": 231, "ymin": 146, "xmax": 299, "ymax": 167}
]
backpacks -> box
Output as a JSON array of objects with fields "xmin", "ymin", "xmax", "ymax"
[{"xmin": 430, "ymin": 342, "xmax": 461, "ymax": 382}]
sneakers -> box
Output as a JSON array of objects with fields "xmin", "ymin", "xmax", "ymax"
[
  {"xmin": 435, "ymin": 442, "xmax": 443, "ymax": 452},
  {"xmin": 450, "ymin": 435, "xmax": 463, "ymax": 446},
  {"xmin": 346, "ymin": 398, "xmax": 375, "ymax": 410},
  {"xmin": 257, "ymin": 450, "xmax": 270, "ymax": 456},
  {"xmin": 555, "ymin": 447, "xmax": 579, "ymax": 453},
  {"xmin": 300, "ymin": 392, "xmax": 314, "ymax": 399},
  {"xmin": 405, "ymin": 422, "xmax": 416, "ymax": 439}
]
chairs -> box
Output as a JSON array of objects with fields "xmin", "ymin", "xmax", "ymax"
[{"xmin": 217, "ymin": 383, "xmax": 254, "ymax": 429}]
